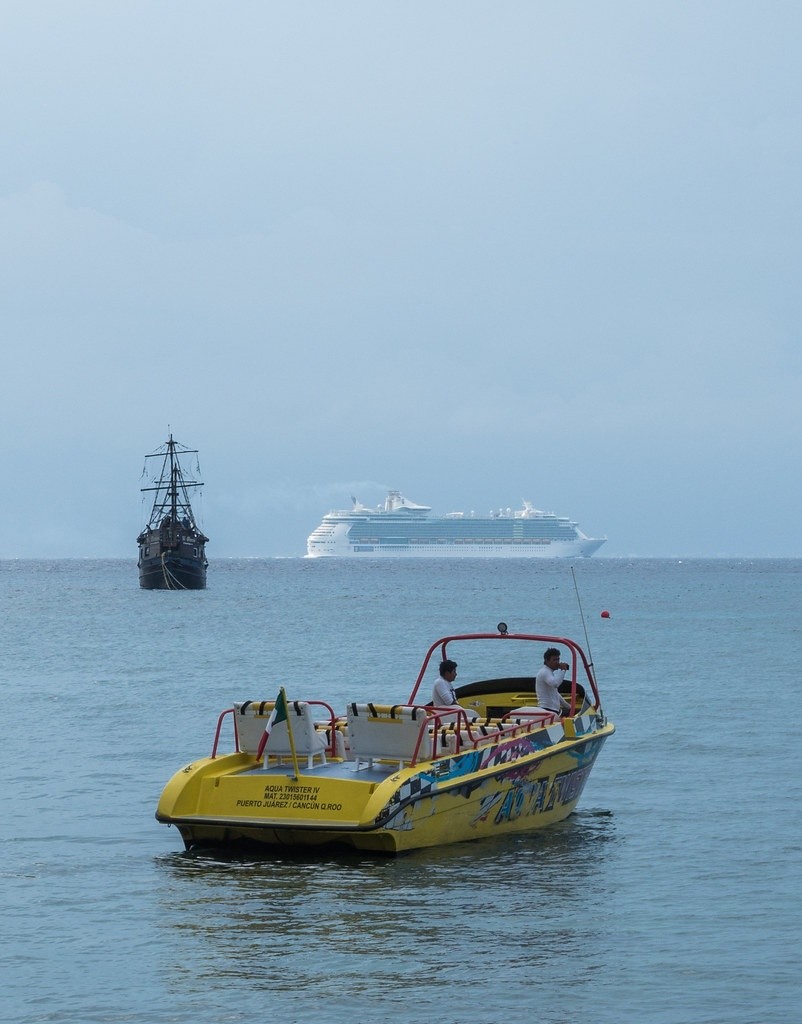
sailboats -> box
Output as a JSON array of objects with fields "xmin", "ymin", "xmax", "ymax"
[{"xmin": 136, "ymin": 424, "xmax": 213, "ymax": 590}]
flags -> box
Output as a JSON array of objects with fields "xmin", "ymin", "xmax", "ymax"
[{"xmin": 255, "ymin": 690, "xmax": 288, "ymax": 762}]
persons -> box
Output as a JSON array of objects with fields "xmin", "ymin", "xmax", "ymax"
[
  {"xmin": 432, "ymin": 660, "xmax": 459, "ymax": 706},
  {"xmin": 536, "ymin": 648, "xmax": 571, "ymax": 714}
]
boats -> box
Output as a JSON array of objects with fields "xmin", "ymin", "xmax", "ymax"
[{"xmin": 153, "ymin": 569, "xmax": 614, "ymax": 859}]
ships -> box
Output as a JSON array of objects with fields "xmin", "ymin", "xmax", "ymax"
[{"xmin": 304, "ymin": 490, "xmax": 609, "ymax": 561}]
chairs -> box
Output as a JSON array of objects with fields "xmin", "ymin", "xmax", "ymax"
[{"xmin": 232, "ymin": 700, "xmax": 563, "ymax": 777}]
